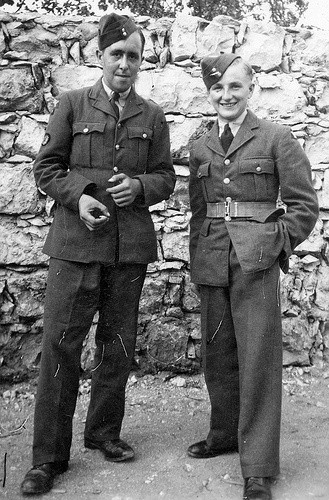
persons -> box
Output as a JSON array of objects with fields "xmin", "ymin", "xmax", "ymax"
[
  {"xmin": 188, "ymin": 55, "xmax": 319, "ymax": 500},
  {"xmin": 21, "ymin": 13, "xmax": 176, "ymax": 495}
]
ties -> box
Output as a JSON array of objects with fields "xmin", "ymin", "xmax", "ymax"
[
  {"xmin": 220, "ymin": 124, "xmax": 234, "ymax": 154},
  {"xmin": 108, "ymin": 92, "xmax": 119, "ymax": 120}
]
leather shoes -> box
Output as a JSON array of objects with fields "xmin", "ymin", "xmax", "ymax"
[
  {"xmin": 187, "ymin": 440, "xmax": 239, "ymax": 458},
  {"xmin": 21, "ymin": 460, "xmax": 69, "ymax": 493},
  {"xmin": 84, "ymin": 436, "xmax": 135, "ymax": 461},
  {"xmin": 243, "ymin": 476, "xmax": 272, "ymax": 500}
]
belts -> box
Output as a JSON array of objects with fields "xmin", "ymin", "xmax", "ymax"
[{"xmin": 206, "ymin": 202, "xmax": 277, "ymax": 217}]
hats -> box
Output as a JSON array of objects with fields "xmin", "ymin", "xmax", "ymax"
[
  {"xmin": 98, "ymin": 12, "xmax": 136, "ymax": 50},
  {"xmin": 201, "ymin": 54, "xmax": 242, "ymax": 90}
]
